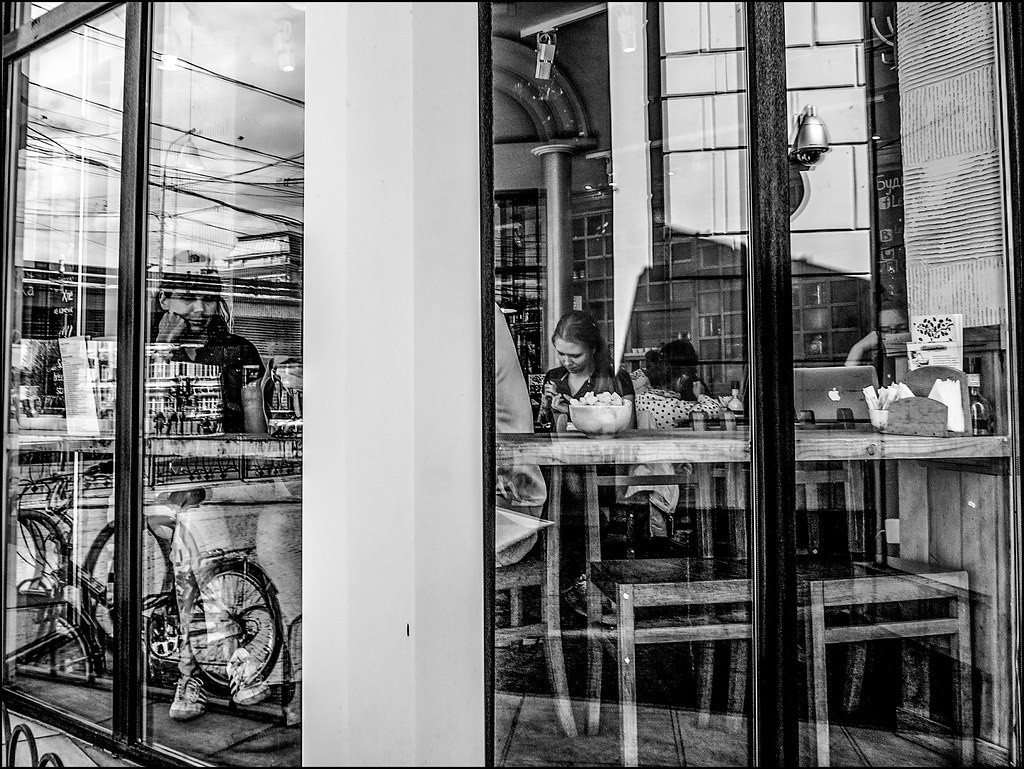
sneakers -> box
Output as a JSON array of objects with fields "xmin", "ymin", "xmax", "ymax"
[
  {"xmin": 225, "ymin": 646, "xmax": 273, "ymax": 705},
  {"xmin": 169, "ymin": 677, "xmax": 208, "ymax": 720}
]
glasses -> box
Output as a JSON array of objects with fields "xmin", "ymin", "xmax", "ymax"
[{"xmin": 876, "ymin": 323, "xmax": 909, "ymax": 341}]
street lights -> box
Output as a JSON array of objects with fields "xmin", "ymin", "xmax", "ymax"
[{"xmin": 157, "ymin": 126, "xmax": 203, "ymax": 288}]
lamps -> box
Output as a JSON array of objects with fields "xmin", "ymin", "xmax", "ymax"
[
  {"xmin": 614, "ymin": 8, "xmax": 636, "ymax": 52},
  {"xmin": 533, "ymin": 28, "xmax": 555, "ymax": 79},
  {"xmin": 272, "ymin": 17, "xmax": 298, "ymax": 72}
]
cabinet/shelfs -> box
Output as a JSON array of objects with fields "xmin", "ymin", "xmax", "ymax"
[{"xmin": 571, "ymin": 174, "xmax": 874, "ymax": 397}]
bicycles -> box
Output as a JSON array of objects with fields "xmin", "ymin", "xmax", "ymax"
[{"xmin": 5, "ymin": 464, "xmax": 285, "ymax": 698}]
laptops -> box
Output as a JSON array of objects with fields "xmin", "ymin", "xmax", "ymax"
[{"xmin": 794, "ymin": 366, "xmax": 880, "ymax": 423}]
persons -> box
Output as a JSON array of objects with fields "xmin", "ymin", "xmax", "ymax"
[
  {"xmin": 537, "ymin": 308, "xmax": 637, "ymax": 616},
  {"xmin": 254, "ymin": 302, "xmax": 550, "ymax": 728},
  {"xmin": 843, "ymin": 296, "xmax": 909, "ymax": 388},
  {"xmin": 152, "ymin": 251, "xmax": 270, "ymax": 717},
  {"xmin": 632, "ymin": 339, "xmax": 724, "ymax": 553}
]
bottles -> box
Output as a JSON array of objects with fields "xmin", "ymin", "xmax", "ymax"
[
  {"xmin": 727, "ymin": 389, "xmax": 745, "ymax": 425},
  {"xmin": 964, "ymin": 374, "xmax": 995, "ymax": 437}
]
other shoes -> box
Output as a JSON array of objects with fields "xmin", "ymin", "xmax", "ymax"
[{"xmin": 282, "ymin": 706, "xmax": 300, "ymax": 727}]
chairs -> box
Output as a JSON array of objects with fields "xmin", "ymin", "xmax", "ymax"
[{"xmin": 496, "ymin": 407, "xmax": 977, "ymax": 767}]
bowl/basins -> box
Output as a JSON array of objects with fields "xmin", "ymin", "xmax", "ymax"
[
  {"xmin": 568, "ymin": 404, "xmax": 633, "ymax": 439},
  {"xmin": 867, "ymin": 408, "xmax": 889, "ymax": 435}
]
